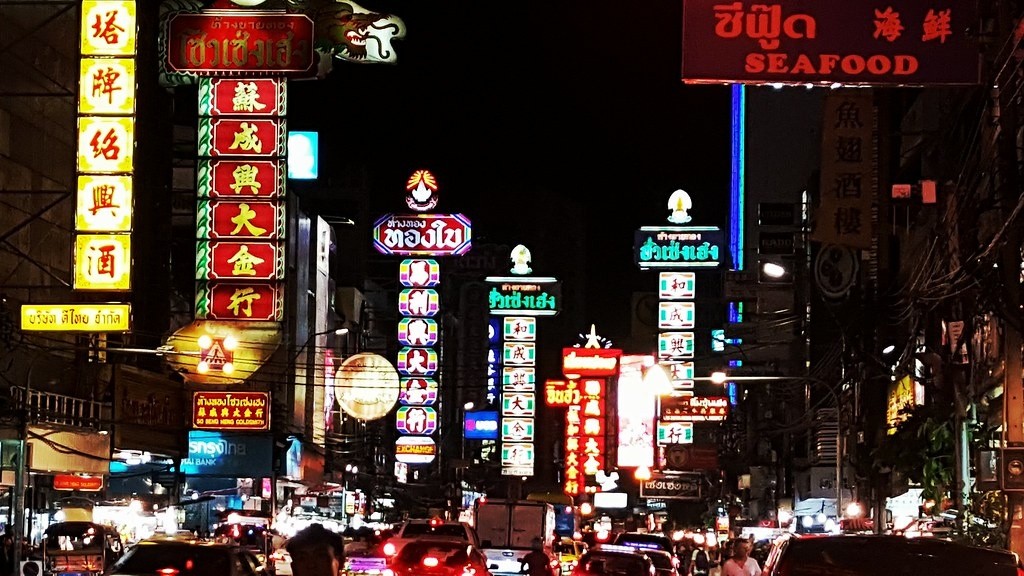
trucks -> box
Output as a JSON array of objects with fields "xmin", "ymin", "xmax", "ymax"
[{"xmin": 474, "ymin": 498, "xmax": 558, "ymax": 570}]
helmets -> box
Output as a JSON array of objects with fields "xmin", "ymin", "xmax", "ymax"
[{"xmin": 531, "ymin": 536, "xmax": 543, "ymax": 543}]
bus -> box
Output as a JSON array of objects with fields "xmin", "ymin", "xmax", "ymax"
[{"xmin": 528, "ymin": 494, "xmax": 579, "ymax": 540}]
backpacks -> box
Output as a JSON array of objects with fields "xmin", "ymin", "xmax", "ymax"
[{"xmin": 695, "ymin": 547, "xmax": 709, "ymax": 571}]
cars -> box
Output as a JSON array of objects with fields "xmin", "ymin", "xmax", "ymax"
[
  {"xmin": 732, "ymin": 517, "xmax": 1020, "ymax": 576},
  {"xmin": 42, "ymin": 515, "xmax": 492, "ymax": 576},
  {"xmin": 558, "ymin": 532, "xmax": 681, "ymax": 576}
]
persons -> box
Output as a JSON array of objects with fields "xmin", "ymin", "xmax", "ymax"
[
  {"xmin": 520, "ymin": 537, "xmax": 554, "ymax": 576},
  {"xmin": 661, "ymin": 521, "xmax": 772, "ymax": 576},
  {"xmin": 287, "ymin": 523, "xmax": 346, "ymax": 576}
]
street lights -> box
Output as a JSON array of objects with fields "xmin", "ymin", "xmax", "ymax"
[
  {"xmin": 760, "ymin": 260, "xmax": 847, "ymax": 455},
  {"xmin": 460, "ymin": 401, "xmax": 475, "ymax": 481},
  {"xmin": 271, "ymin": 326, "xmax": 348, "ymax": 524}
]
